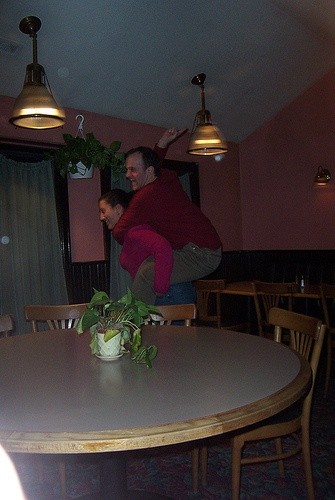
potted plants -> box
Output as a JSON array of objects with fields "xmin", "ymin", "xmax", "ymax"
[
  {"xmin": 76, "ymin": 290, "xmax": 157, "ymax": 368},
  {"xmin": 51, "ymin": 132, "xmax": 128, "ymax": 187}
]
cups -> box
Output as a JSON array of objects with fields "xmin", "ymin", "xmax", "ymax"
[{"xmin": 295, "ymin": 274, "xmax": 311, "ymax": 288}]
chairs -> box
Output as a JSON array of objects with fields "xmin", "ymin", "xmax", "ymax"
[{"xmin": 0, "ymin": 272, "xmax": 335, "ymax": 500}]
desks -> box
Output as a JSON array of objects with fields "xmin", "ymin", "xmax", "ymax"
[
  {"xmin": 210, "ymin": 283, "xmax": 335, "ymax": 322},
  {"xmin": 0, "ymin": 324, "xmax": 313, "ymax": 500}
]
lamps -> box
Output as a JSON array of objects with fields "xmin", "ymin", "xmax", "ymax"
[
  {"xmin": 185, "ymin": 73, "xmax": 226, "ymax": 155},
  {"xmin": 8, "ymin": 15, "xmax": 65, "ymax": 129},
  {"xmin": 313, "ymin": 166, "xmax": 331, "ymax": 187}
]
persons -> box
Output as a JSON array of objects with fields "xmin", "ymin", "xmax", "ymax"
[
  {"xmin": 112, "ymin": 146, "xmax": 223, "ymax": 307},
  {"xmin": 98, "ymin": 188, "xmax": 197, "ymax": 326}
]
